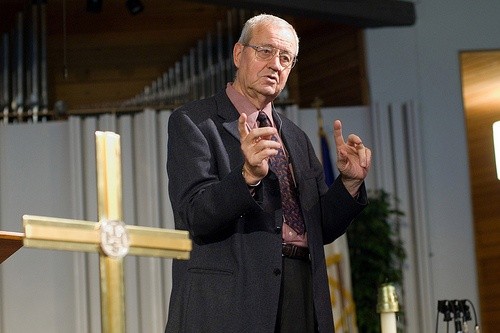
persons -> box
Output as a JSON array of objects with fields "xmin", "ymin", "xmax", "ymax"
[{"xmin": 164, "ymin": 13, "xmax": 373, "ymax": 333}]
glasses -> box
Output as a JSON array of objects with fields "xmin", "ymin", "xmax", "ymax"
[{"xmin": 244, "ymin": 44, "xmax": 298, "ymax": 68}]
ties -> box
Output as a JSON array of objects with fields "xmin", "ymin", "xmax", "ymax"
[{"xmin": 256, "ymin": 111, "xmax": 306, "ymax": 236}]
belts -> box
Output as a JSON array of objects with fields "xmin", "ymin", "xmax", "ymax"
[{"xmin": 282, "ymin": 243, "xmax": 310, "ymax": 260}]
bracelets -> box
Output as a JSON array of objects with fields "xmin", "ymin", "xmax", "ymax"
[{"xmin": 243, "ymin": 180, "xmax": 263, "ymax": 189}]
